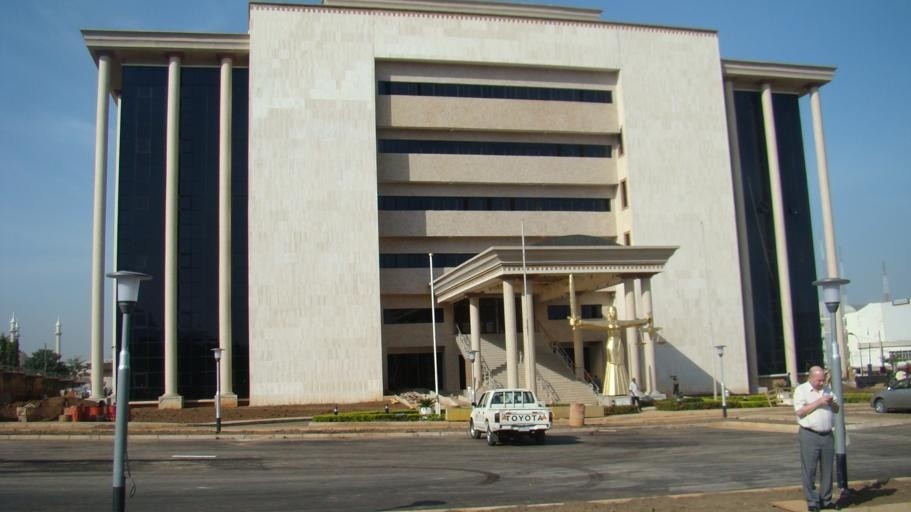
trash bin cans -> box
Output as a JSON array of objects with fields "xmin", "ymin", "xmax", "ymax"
[{"xmin": 570, "ymin": 403, "xmax": 585, "ymax": 426}]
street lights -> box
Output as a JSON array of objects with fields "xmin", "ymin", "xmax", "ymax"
[
  {"xmin": 811, "ymin": 276, "xmax": 854, "ymax": 498},
  {"xmin": 106, "ymin": 270, "xmax": 152, "ymax": 512},
  {"xmin": 848, "ymin": 331, "xmax": 864, "ymax": 377},
  {"xmin": 713, "ymin": 344, "xmax": 729, "ymax": 417},
  {"xmin": 210, "ymin": 347, "xmax": 227, "ymax": 432}
]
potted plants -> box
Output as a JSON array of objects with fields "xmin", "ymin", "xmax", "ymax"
[{"xmin": 416, "ymin": 398, "xmax": 437, "ymax": 415}]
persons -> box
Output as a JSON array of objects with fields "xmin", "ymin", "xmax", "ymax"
[
  {"xmin": 566, "ymin": 303, "xmax": 650, "ymax": 396},
  {"xmin": 629, "ymin": 377, "xmax": 643, "ymax": 412},
  {"xmin": 793, "ymin": 365, "xmax": 840, "ymax": 512},
  {"xmin": 672, "ymin": 377, "xmax": 680, "ymax": 396},
  {"xmin": 568, "ymin": 360, "xmax": 601, "ymax": 391}
]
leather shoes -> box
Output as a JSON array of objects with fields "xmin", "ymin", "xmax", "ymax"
[
  {"xmin": 809, "ymin": 506, "xmax": 819, "ymax": 512},
  {"xmin": 823, "ymin": 503, "xmax": 841, "ymax": 509}
]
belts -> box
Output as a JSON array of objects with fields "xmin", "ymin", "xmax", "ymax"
[{"xmin": 800, "ymin": 427, "xmax": 831, "ymax": 435}]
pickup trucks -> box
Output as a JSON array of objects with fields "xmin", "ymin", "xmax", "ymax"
[{"xmin": 468, "ymin": 388, "xmax": 554, "ymax": 445}]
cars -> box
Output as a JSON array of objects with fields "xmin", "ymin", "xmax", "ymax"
[
  {"xmin": 870, "ymin": 377, "xmax": 911, "ymax": 413},
  {"xmin": 81, "ymin": 385, "xmax": 92, "ymax": 397}
]
